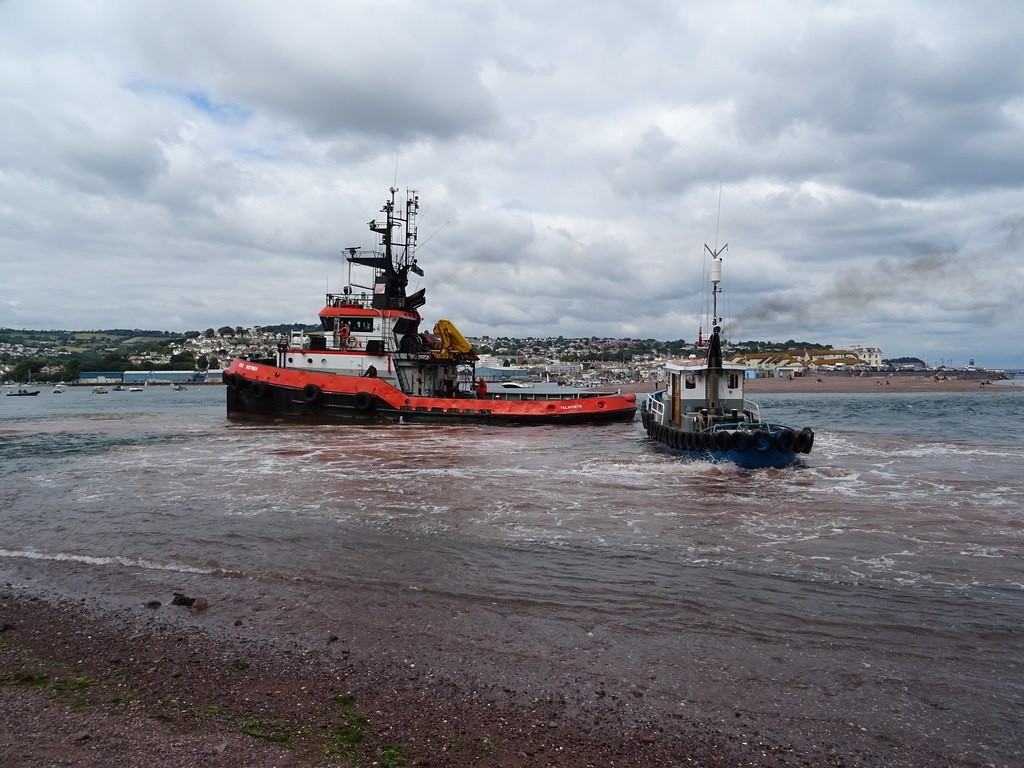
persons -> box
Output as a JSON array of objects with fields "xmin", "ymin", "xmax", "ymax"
[
  {"xmin": 361, "ymin": 362, "xmax": 377, "ymax": 378},
  {"xmin": 477, "ymin": 377, "xmax": 487, "ymax": 413},
  {"xmin": 655, "ymin": 381, "xmax": 658, "ymax": 390},
  {"xmin": 339, "ymin": 322, "xmax": 350, "ymax": 350}
]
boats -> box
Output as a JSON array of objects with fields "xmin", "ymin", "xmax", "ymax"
[
  {"xmin": 129, "ymin": 387, "xmax": 143, "ymax": 391},
  {"xmin": 93, "ymin": 386, "xmax": 108, "ymax": 394},
  {"xmin": 224, "ymin": 155, "xmax": 637, "ymax": 426},
  {"xmin": 6, "ymin": 385, "xmax": 40, "ymax": 396},
  {"xmin": 112, "ymin": 384, "xmax": 126, "ymax": 391},
  {"xmin": 174, "ymin": 386, "xmax": 186, "ymax": 391},
  {"xmin": 53, "ymin": 385, "xmax": 65, "ymax": 393},
  {"xmin": 641, "ymin": 182, "xmax": 815, "ymax": 470}
]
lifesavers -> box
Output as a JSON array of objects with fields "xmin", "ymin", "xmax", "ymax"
[
  {"xmin": 250, "ymin": 381, "xmax": 267, "ymax": 399},
  {"xmin": 641, "ymin": 401, "xmax": 674, "ymax": 449},
  {"xmin": 228, "ymin": 373, "xmax": 242, "ymax": 390},
  {"xmin": 692, "ymin": 433, "xmax": 702, "ymax": 451},
  {"xmin": 673, "ymin": 431, "xmax": 680, "ymax": 450},
  {"xmin": 714, "ymin": 431, "xmax": 730, "ymax": 452},
  {"xmin": 345, "ymin": 335, "xmax": 358, "ymax": 347},
  {"xmin": 751, "ymin": 431, "xmax": 774, "ymax": 453},
  {"xmin": 731, "ymin": 431, "xmax": 751, "ymax": 453},
  {"xmin": 793, "ymin": 426, "xmax": 814, "ymax": 454},
  {"xmin": 222, "ymin": 371, "xmax": 228, "ymax": 385},
  {"xmin": 679, "ymin": 431, "xmax": 687, "ymax": 451},
  {"xmin": 685, "ymin": 432, "xmax": 694, "ymax": 451},
  {"xmin": 353, "ymin": 391, "xmax": 373, "ymax": 411},
  {"xmin": 774, "ymin": 429, "xmax": 796, "ymax": 452},
  {"xmin": 302, "ymin": 383, "xmax": 321, "ymax": 403},
  {"xmin": 708, "ymin": 435, "xmax": 717, "ymax": 453},
  {"xmin": 702, "ymin": 432, "xmax": 712, "ymax": 450}
]
